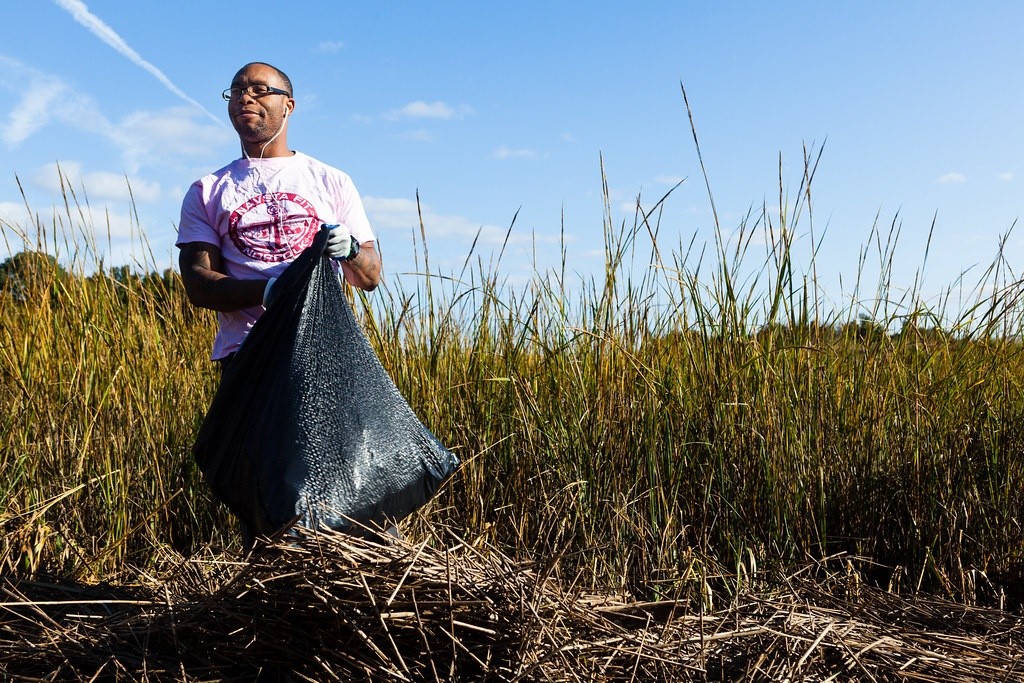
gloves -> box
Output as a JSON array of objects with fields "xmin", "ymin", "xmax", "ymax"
[{"xmin": 326, "ymin": 223, "xmax": 351, "ymax": 260}]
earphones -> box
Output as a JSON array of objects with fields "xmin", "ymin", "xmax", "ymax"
[{"xmin": 284, "ymin": 106, "xmax": 289, "ymax": 119}]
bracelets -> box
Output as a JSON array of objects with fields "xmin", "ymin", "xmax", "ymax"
[{"xmin": 263, "ymin": 277, "xmax": 278, "ymax": 307}]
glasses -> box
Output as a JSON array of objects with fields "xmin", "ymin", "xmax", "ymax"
[{"xmin": 222, "ymin": 83, "xmax": 291, "ymax": 101}]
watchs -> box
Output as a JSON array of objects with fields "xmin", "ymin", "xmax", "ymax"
[{"xmin": 344, "ymin": 234, "xmax": 361, "ymax": 263}]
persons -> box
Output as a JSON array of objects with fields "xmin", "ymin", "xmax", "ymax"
[{"xmin": 175, "ymin": 61, "xmax": 404, "ymax": 566}]
